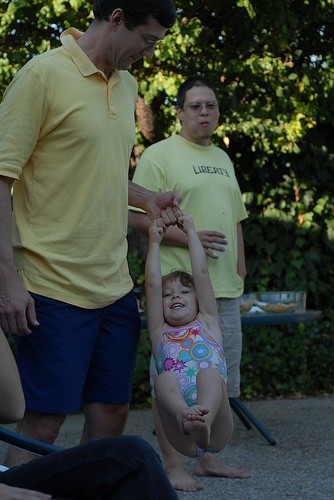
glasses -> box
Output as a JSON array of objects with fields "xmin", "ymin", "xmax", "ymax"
[
  {"xmin": 126, "ymin": 16, "xmax": 163, "ymax": 49},
  {"xmin": 179, "ymin": 101, "xmax": 221, "ymax": 113}
]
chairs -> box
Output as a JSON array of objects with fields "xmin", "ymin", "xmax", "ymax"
[{"xmin": 0, "ymin": 425, "xmax": 182, "ymax": 500}]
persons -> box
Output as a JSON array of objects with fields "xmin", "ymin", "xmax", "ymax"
[
  {"xmin": 1, "ymin": 2, "xmax": 183, "ymax": 470},
  {"xmin": 144, "ymin": 210, "xmax": 236, "ymax": 459},
  {"xmin": 128, "ymin": 76, "xmax": 250, "ymax": 492},
  {"xmin": 1, "ymin": 327, "xmax": 182, "ymax": 500}
]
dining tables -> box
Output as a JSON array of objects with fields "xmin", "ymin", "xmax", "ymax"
[{"xmin": 139, "ymin": 307, "xmax": 323, "ymax": 447}]
